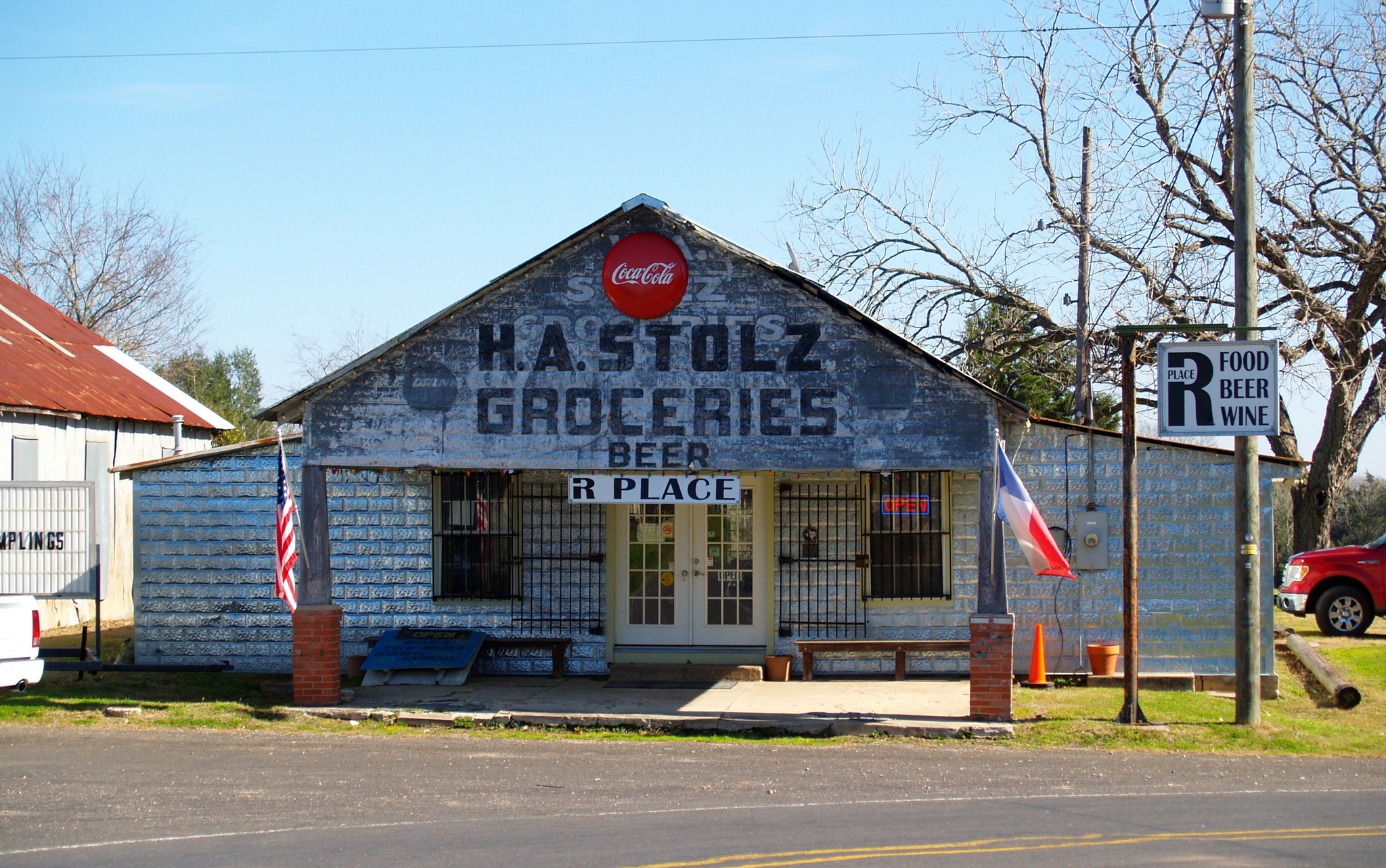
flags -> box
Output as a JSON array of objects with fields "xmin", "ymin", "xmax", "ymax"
[
  {"xmin": 994, "ymin": 439, "xmax": 1077, "ymax": 582},
  {"xmin": 476, "ymin": 482, "xmax": 488, "ymax": 583},
  {"xmin": 274, "ymin": 443, "xmax": 298, "ymax": 614}
]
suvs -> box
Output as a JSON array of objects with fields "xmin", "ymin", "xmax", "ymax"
[{"xmin": 1275, "ymin": 532, "xmax": 1386, "ymax": 639}]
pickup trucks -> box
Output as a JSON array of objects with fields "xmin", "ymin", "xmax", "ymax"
[{"xmin": 0, "ymin": 594, "xmax": 45, "ymax": 693}]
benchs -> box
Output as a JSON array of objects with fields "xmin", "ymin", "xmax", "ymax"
[
  {"xmin": 794, "ymin": 640, "xmax": 970, "ymax": 681},
  {"xmin": 483, "ymin": 637, "xmax": 571, "ymax": 678}
]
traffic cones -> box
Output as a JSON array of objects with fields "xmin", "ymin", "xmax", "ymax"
[{"xmin": 1019, "ymin": 624, "xmax": 1056, "ymax": 690}]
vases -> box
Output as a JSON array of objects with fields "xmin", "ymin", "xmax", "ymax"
[
  {"xmin": 1087, "ymin": 643, "xmax": 1120, "ymax": 676},
  {"xmin": 764, "ymin": 654, "xmax": 792, "ymax": 682},
  {"xmin": 346, "ymin": 655, "xmax": 367, "ymax": 680}
]
